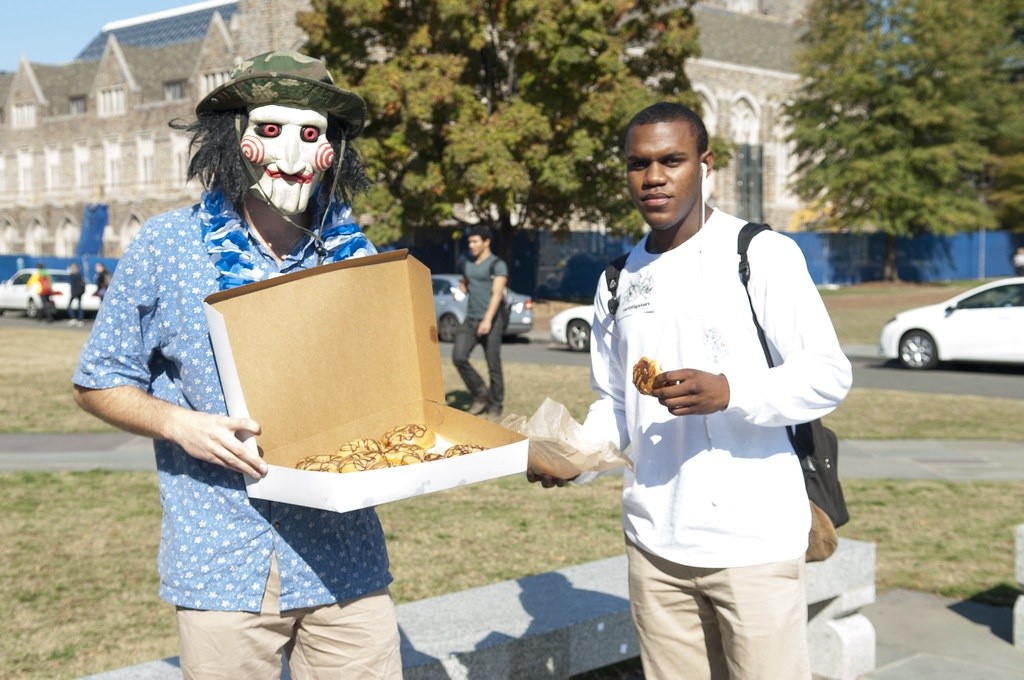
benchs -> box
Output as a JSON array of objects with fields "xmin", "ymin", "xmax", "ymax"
[{"xmin": 83, "ymin": 538, "xmax": 876, "ymax": 680}]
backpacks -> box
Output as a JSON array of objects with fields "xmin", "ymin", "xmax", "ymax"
[
  {"xmin": 462, "ymin": 258, "xmax": 511, "ymax": 330},
  {"xmin": 605, "ymin": 222, "xmax": 849, "ymax": 529}
]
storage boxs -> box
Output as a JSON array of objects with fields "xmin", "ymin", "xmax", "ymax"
[{"xmin": 203, "ymin": 247, "xmax": 530, "ymax": 512}]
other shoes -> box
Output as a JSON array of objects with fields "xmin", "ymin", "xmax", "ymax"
[{"xmin": 474, "ymin": 403, "xmax": 503, "ymax": 416}]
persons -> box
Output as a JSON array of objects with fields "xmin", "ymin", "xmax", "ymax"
[
  {"xmin": 524, "ymin": 102, "xmax": 852, "ymax": 680},
  {"xmin": 24, "ymin": 263, "xmax": 57, "ymax": 323},
  {"xmin": 64, "ymin": 263, "xmax": 85, "ymax": 327},
  {"xmin": 68, "ymin": 49, "xmax": 405, "ymax": 680},
  {"xmin": 450, "ymin": 225, "xmax": 510, "ymax": 421},
  {"xmin": 1011, "ymin": 246, "xmax": 1024, "ymax": 277},
  {"xmin": 92, "ymin": 262, "xmax": 113, "ymax": 302}
]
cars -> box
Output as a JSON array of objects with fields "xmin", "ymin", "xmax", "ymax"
[
  {"xmin": 877, "ymin": 275, "xmax": 1024, "ymax": 369},
  {"xmin": 430, "ymin": 273, "xmax": 533, "ymax": 342},
  {"xmin": 549, "ymin": 304, "xmax": 595, "ymax": 351},
  {"xmin": 0, "ymin": 269, "xmax": 102, "ymax": 318}
]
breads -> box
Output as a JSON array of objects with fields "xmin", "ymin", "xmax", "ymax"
[
  {"xmin": 291, "ymin": 424, "xmax": 493, "ymax": 476},
  {"xmin": 632, "ymin": 357, "xmax": 676, "ymax": 395}
]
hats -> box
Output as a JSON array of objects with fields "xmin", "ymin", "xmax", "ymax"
[{"xmin": 195, "ymin": 50, "xmax": 367, "ymax": 141}]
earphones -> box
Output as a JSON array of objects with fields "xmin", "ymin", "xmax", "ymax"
[{"xmin": 701, "ymin": 162, "xmax": 708, "ymax": 176}]
